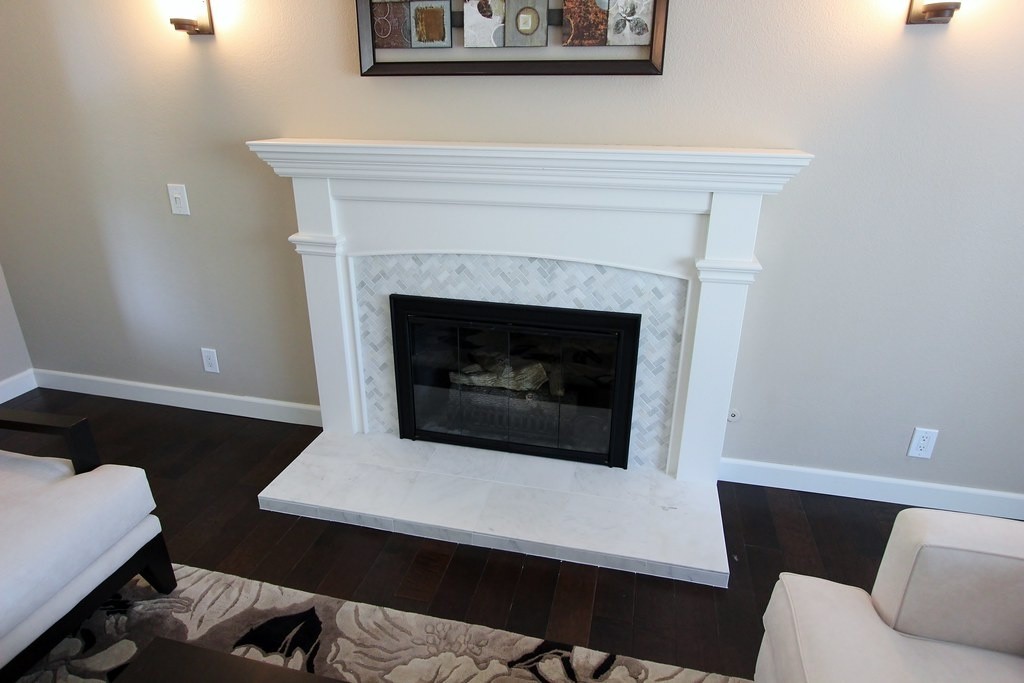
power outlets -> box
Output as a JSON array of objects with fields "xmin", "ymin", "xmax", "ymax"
[
  {"xmin": 200, "ymin": 347, "xmax": 220, "ymax": 373},
  {"xmin": 906, "ymin": 428, "xmax": 939, "ymax": 459}
]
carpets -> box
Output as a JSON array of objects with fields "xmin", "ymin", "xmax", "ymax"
[{"xmin": 0, "ymin": 561, "xmax": 755, "ymax": 683}]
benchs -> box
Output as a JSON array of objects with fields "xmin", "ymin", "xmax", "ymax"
[{"xmin": 0, "ymin": 408, "xmax": 178, "ymax": 683}]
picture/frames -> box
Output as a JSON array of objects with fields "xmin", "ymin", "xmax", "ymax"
[{"xmin": 355, "ymin": 0, "xmax": 669, "ymax": 77}]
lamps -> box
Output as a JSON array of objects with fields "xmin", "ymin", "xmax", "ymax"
[
  {"xmin": 906, "ymin": 0, "xmax": 961, "ymax": 24},
  {"xmin": 169, "ymin": 0, "xmax": 214, "ymax": 35}
]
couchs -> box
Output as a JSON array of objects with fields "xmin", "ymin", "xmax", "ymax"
[{"xmin": 754, "ymin": 508, "xmax": 1024, "ymax": 683}]
